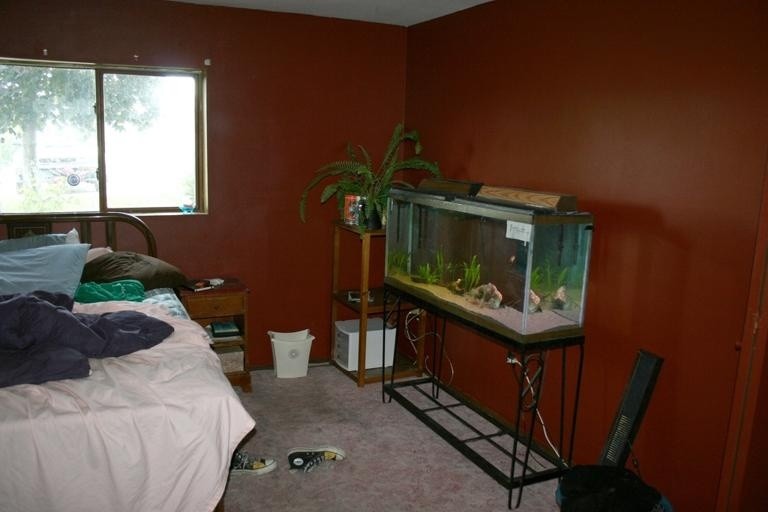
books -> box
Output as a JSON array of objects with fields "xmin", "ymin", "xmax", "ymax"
[{"xmin": 211, "ymin": 321, "xmax": 241, "ymax": 337}]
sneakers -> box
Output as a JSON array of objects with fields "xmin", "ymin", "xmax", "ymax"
[
  {"xmin": 287, "ymin": 446, "xmax": 346, "ymax": 474},
  {"xmin": 229, "ymin": 452, "xmax": 277, "ymax": 476}
]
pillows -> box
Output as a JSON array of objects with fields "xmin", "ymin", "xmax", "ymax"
[{"xmin": 0, "ymin": 228, "xmax": 196, "ymax": 299}]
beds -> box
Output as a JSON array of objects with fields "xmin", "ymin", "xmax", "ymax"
[{"xmin": 0, "ymin": 213, "xmax": 236, "ymax": 512}]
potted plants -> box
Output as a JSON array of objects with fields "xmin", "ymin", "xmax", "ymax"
[{"xmin": 298, "ymin": 121, "xmax": 447, "ymax": 238}]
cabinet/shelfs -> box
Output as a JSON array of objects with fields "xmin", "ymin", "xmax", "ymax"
[
  {"xmin": 330, "ymin": 221, "xmax": 428, "ymax": 387},
  {"xmin": 380, "ymin": 283, "xmax": 587, "ymax": 510},
  {"xmin": 175, "ymin": 275, "xmax": 252, "ymax": 393}
]
